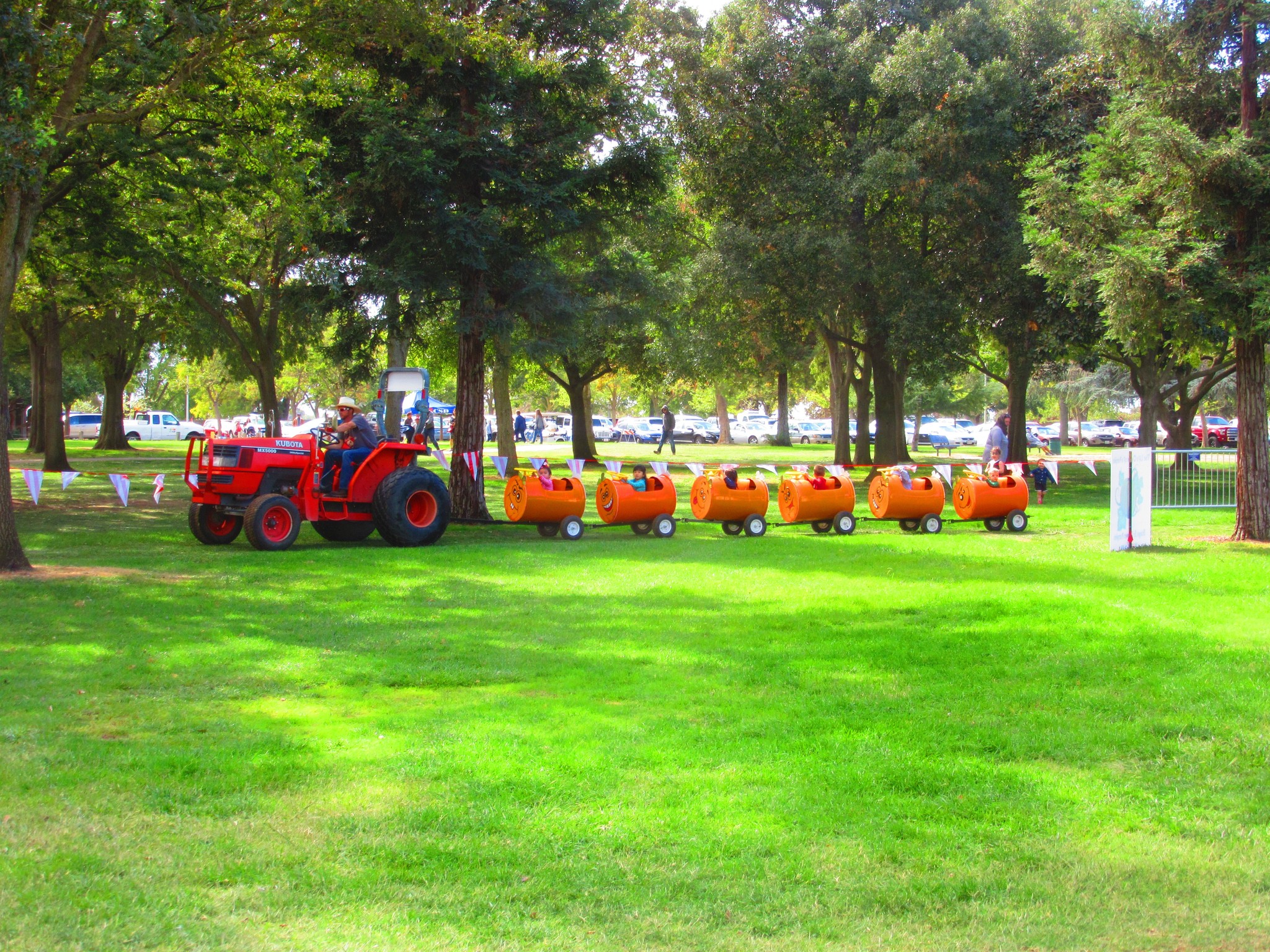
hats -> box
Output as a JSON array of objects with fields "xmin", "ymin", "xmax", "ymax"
[
  {"xmin": 540, "ymin": 459, "xmax": 549, "ymax": 469},
  {"xmin": 1026, "ymin": 427, "xmax": 1032, "ymax": 430},
  {"xmin": 331, "ymin": 397, "xmax": 362, "ymax": 413},
  {"xmin": 415, "ymin": 417, "xmax": 419, "ymax": 421}
]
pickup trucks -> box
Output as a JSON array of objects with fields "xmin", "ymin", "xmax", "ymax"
[{"xmin": 96, "ymin": 410, "xmax": 206, "ymax": 441}]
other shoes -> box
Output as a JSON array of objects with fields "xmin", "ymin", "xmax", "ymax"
[
  {"xmin": 515, "ymin": 440, "xmax": 543, "ymax": 444},
  {"xmin": 654, "ymin": 450, "xmax": 660, "ymax": 455},
  {"xmin": 329, "ymin": 491, "xmax": 347, "ymax": 498},
  {"xmin": 671, "ymin": 453, "xmax": 676, "ymax": 456},
  {"xmin": 319, "ymin": 487, "xmax": 330, "ymax": 494},
  {"xmin": 1046, "ymin": 452, "xmax": 1056, "ymax": 456},
  {"xmin": 1038, "ymin": 499, "xmax": 1043, "ymax": 504},
  {"xmin": 449, "ymin": 448, "xmax": 452, "ymax": 452}
]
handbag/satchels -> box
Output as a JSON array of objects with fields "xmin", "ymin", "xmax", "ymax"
[
  {"xmin": 447, "ymin": 425, "xmax": 454, "ymax": 433},
  {"xmin": 542, "ymin": 417, "xmax": 548, "ymax": 428}
]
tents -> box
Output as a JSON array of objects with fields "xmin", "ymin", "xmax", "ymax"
[{"xmin": 383, "ymin": 390, "xmax": 456, "ymax": 441}]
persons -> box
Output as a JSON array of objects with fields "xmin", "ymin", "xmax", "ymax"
[
  {"xmin": 485, "ymin": 420, "xmax": 493, "ymax": 442},
  {"xmin": 535, "ymin": 466, "xmax": 553, "ymax": 491},
  {"xmin": 984, "ymin": 446, "xmax": 1004, "ymax": 476},
  {"xmin": 422, "ymin": 410, "xmax": 440, "ymax": 451},
  {"xmin": 619, "ymin": 464, "xmax": 647, "ymax": 492},
  {"xmin": 719, "ymin": 468, "xmax": 738, "ymax": 490},
  {"xmin": 1028, "ymin": 459, "xmax": 1054, "ymax": 504},
  {"xmin": 514, "ymin": 410, "xmax": 527, "ymax": 443},
  {"xmin": 404, "ymin": 412, "xmax": 413, "ymax": 426},
  {"xmin": 414, "ymin": 417, "xmax": 419, "ymax": 425},
  {"xmin": 248, "ymin": 431, "xmax": 254, "ymax": 438},
  {"xmin": 511, "ymin": 417, "xmax": 515, "ymax": 441},
  {"xmin": 981, "ymin": 467, "xmax": 1000, "ymax": 488},
  {"xmin": 801, "ymin": 464, "xmax": 826, "ymax": 491},
  {"xmin": 293, "ymin": 414, "xmax": 304, "ymax": 427},
  {"xmin": 448, "ymin": 408, "xmax": 456, "ymax": 451},
  {"xmin": 234, "ymin": 420, "xmax": 244, "ymax": 438},
  {"xmin": 653, "ymin": 405, "xmax": 676, "ymax": 455},
  {"xmin": 243, "ymin": 418, "xmax": 251, "ymax": 434},
  {"xmin": 313, "ymin": 397, "xmax": 378, "ymax": 498},
  {"xmin": 530, "ymin": 409, "xmax": 545, "ymax": 444},
  {"xmin": 887, "ymin": 466, "xmax": 912, "ymax": 490},
  {"xmin": 1026, "ymin": 427, "xmax": 1057, "ymax": 455},
  {"xmin": 982, "ymin": 412, "xmax": 1011, "ymax": 463}
]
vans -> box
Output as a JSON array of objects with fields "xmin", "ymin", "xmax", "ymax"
[{"xmin": 62, "ymin": 413, "xmax": 103, "ymax": 438}]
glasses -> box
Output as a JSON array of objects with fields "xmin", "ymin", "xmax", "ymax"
[{"xmin": 337, "ymin": 408, "xmax": 349, "ymax": 412}]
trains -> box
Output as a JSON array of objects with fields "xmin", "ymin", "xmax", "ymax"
[{"xmin": 183, "ymin": 360, "xmax": 1033, "ymax": 548}]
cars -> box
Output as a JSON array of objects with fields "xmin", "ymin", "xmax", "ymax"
[{"xmin": 206, "ymin": 410, "xmax": 1235, "ymax": 450}]
